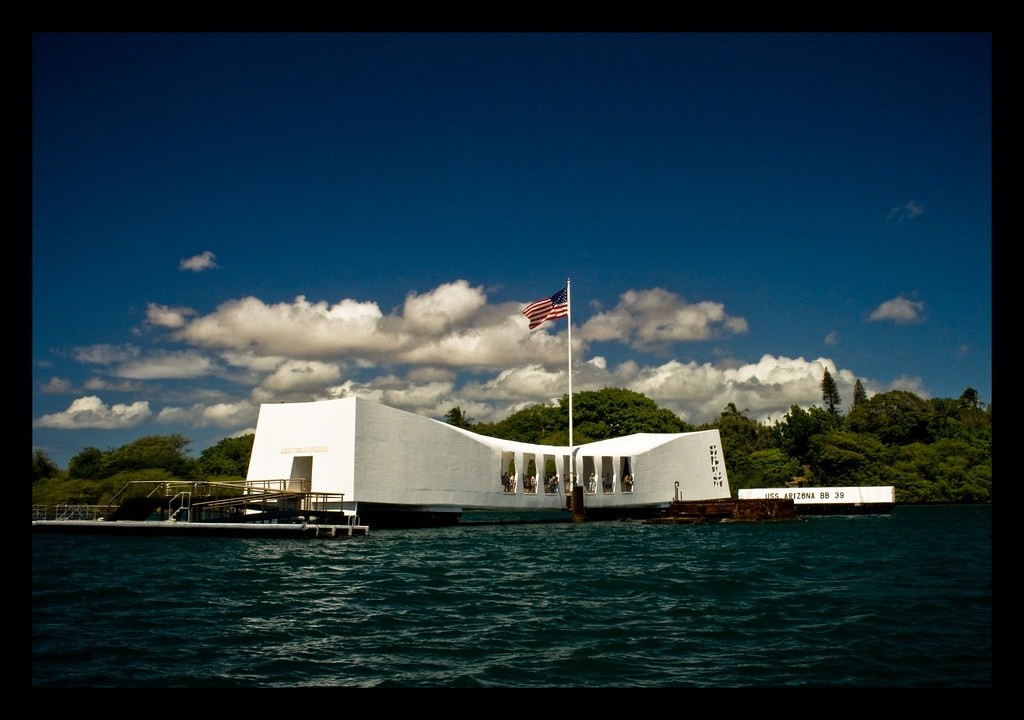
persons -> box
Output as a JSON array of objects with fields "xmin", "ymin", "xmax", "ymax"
[
  {"xmin": 624, "ymin": 474, "xmax": 632, "ymax": 492},
  {"xmin": 522, "ymin": 474, "xmax": 536, "ymax": 493},
  {"xmin": 501, "ymin": 472, "xmax": 515, "ymax": 492},
  {"xmin": 548, "ymin": 476, "xmax": 558, "ymax": 493}
]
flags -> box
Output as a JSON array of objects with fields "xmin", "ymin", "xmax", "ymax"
[{"xmin": 522, "ymin": 287, "xmax": 568, "ymax": 331}]
